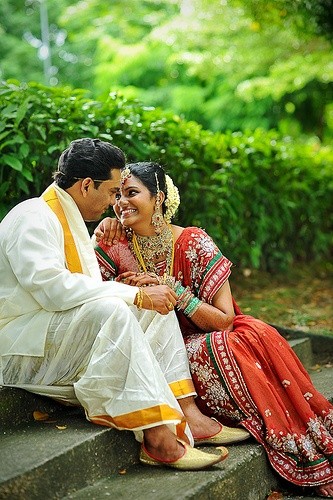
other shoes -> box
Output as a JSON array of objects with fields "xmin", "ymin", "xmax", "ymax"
[
  {"xmin": 193, "ymin": 417, "xmax": 250, "ymax": 447},
  {"xmin": 139, "ymin": 437, "xmax": 229, "ymax": 470}
]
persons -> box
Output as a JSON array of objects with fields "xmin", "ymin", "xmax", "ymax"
[
  {"xmin": 0, "ymin": 138, "xmax": 255, "ymax": 469},
  {"xmin": 89, "ymin": 160, "xmax": 333, "ymax": 500}
]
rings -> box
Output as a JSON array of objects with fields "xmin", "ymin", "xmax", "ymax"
[{"xmin": 168, "ymin": 302, "xmax": 173, "ymax": 308}]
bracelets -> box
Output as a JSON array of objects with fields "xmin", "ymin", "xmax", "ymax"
[
  {"xmin": 151, "ymin": 272, "xmax": 203, "ymax": 319},
  {"xmin": 137, "ymin": 286, "xmax": 143, "ymax": 311}
]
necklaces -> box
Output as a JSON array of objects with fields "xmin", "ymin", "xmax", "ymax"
[{"xmin": 131, "ymin": 227, "xmax": 174, "ymax": 277}]
